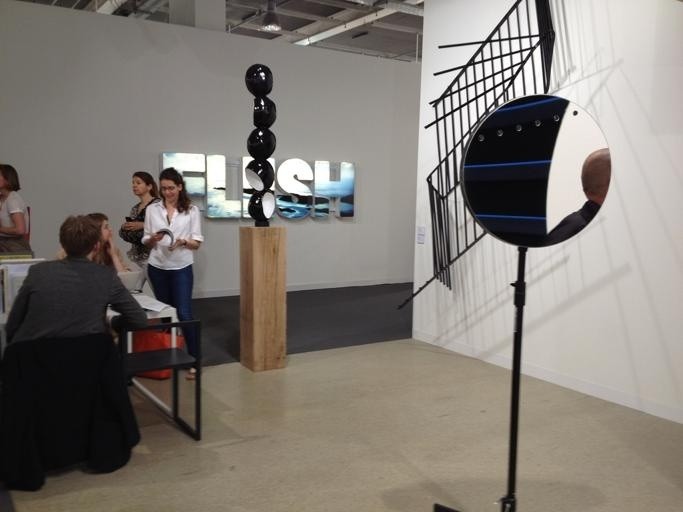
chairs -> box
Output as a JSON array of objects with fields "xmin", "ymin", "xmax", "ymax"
[
  {"xmin": 117, "ymin": 320, "xmax": 203, "ymax": 442},
  {"xmin": 0, "ymin": 332, "xmax": 142, "ymax": 492}
]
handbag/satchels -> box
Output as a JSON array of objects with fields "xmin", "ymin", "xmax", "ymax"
[{"xmin": 118, "ymin": 219, "xmax": 144, "ymax": 245}]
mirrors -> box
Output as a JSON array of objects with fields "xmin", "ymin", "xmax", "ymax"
[{"xmin": 458, "ymin": 93, "xmax": 613, "ymax": 249}]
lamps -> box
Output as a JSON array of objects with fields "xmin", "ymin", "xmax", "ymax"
[{"xmin": 260, "ymin": 1, "xmax": 283, "ymax": 32}]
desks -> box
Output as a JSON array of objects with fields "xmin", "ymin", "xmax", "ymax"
[{"xmin": 105, "ymin": 292, "xmax": 179, "ymax": 419}]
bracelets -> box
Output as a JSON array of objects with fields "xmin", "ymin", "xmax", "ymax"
[{"xmin": 183, "ymin": 239, "xmax": 189, "ymax": 249}]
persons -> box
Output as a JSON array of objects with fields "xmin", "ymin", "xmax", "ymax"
[
  {"xmin": 120, "ymin": 170, "xmax": 162, "ymax": 264},
  {"xmin": 55, "ymin": 213, "xmax": 125, "ymax": 273},
  {"xmin": 1, "ymin": 165, "xmax": 35, "ymax": 257},
  {"xmin": 141, "ymin": 165, "xmax": 203, "ymax": 378},
  {"xmin": 5, "ymin": 212, "xmax": 148, "ymax": 343},
  {"xmin": 542, "ymin": 147, "xmax": 610, "ymax": 247}
]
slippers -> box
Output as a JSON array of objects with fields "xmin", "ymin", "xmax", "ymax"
[{"xmin": 185, "ymin": 371, "xmax": 197, "ymax": 379}]
